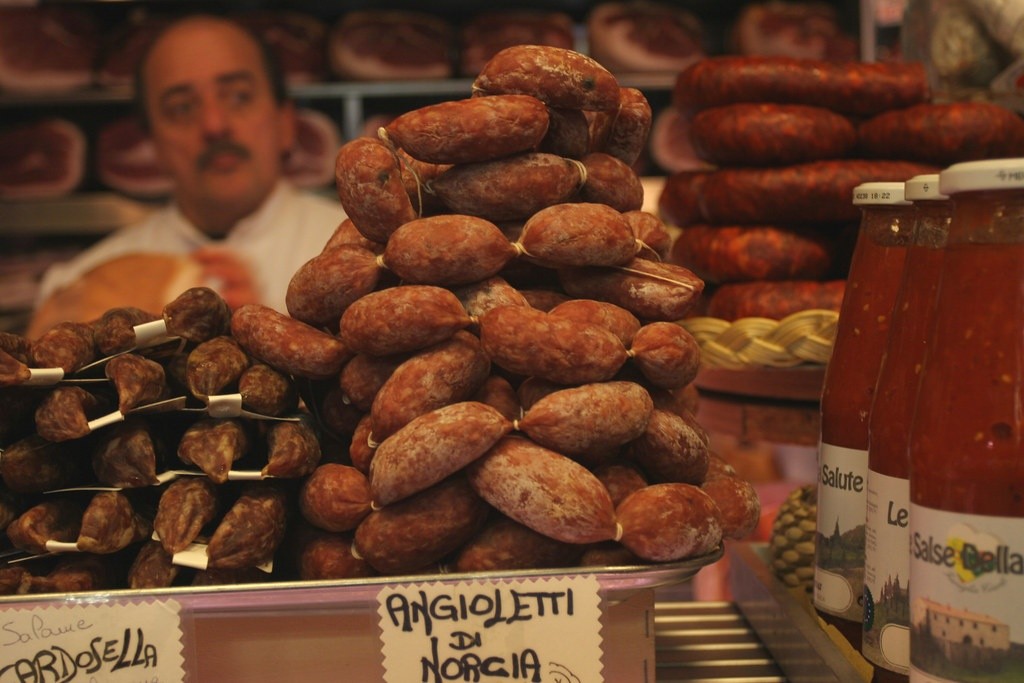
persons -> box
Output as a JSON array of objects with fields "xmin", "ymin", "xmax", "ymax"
[{"xmin": 27, "ymin": 14, "xmax": 348, "ymax": 344}]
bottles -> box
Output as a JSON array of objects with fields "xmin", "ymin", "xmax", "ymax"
[
  {"xmin": 910, "ymin": 158, "xmax": 1024, "ymax": 682},
  {"xmin": 814, "ymin": 181, "xmax": 918, "ymax": 628},
  {"xmin": 861, "ymin": 172, "xmax": 950, "ymax": 677}
]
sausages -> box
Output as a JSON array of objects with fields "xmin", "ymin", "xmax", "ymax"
[{"xmin": 0, "ymin": 42, "xmax": 1023, "ymax": 603}]
